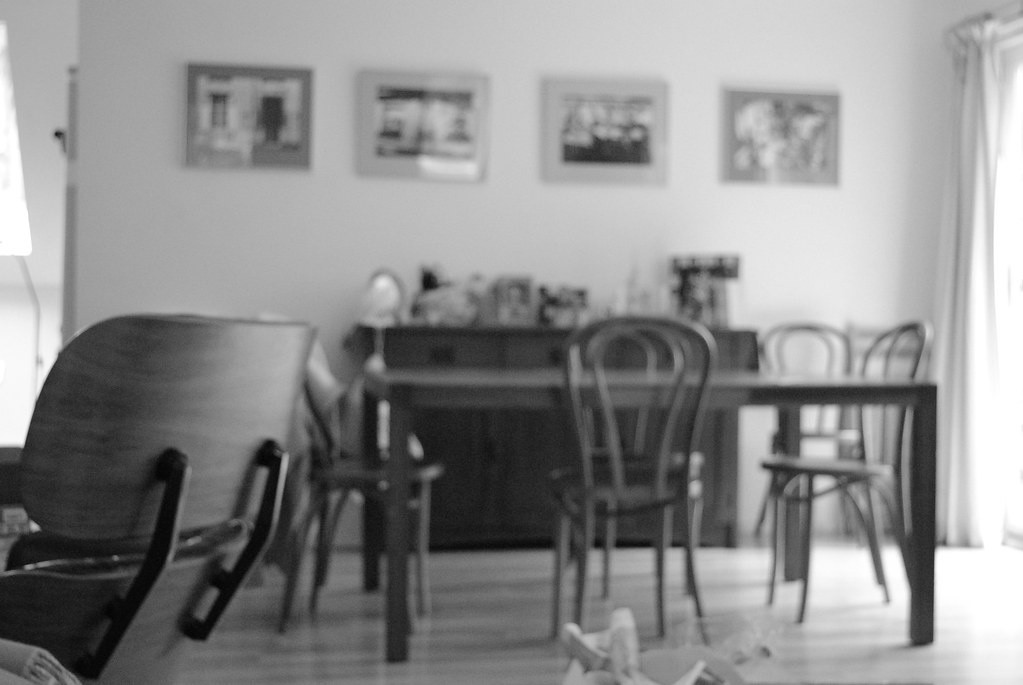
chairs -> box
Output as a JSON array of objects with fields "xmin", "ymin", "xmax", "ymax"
[
  {"xmin": 273, "ymin": 338, "xmax": 444, "ymax": 636},
  {"xmin": 755, "ymin": 321, "xmax": 931, "ymax": 625},
  {"xmin": 542, "ymin": 315, "xmax": 711, "ymax": 651},
  {"xmin": 0, "ymin": 300, "xmax": 319, "ymax": 685}
]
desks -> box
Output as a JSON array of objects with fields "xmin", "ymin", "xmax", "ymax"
[
  {"xmin": 340, "ymin": 325, "xmax": 763, "ymax": 549},
  {"xmin": 362, "ymin": 369, "xmax": 938, "ymax": 666}
]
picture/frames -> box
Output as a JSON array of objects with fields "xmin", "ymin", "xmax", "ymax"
[
  {"xmin": 542, "ymin": 77, "xmax": 670, "ymax": 185},
  {"xmin": 719, "ymin": 88, "xmax": 842, "ymax": 186},
  {"xmin": 185, "ymin": 62, "xmax": 312, "ymax": 170},
  {"xmin": 358, "ymin": 71, "xmax": 494, "ymax": 182}
]
